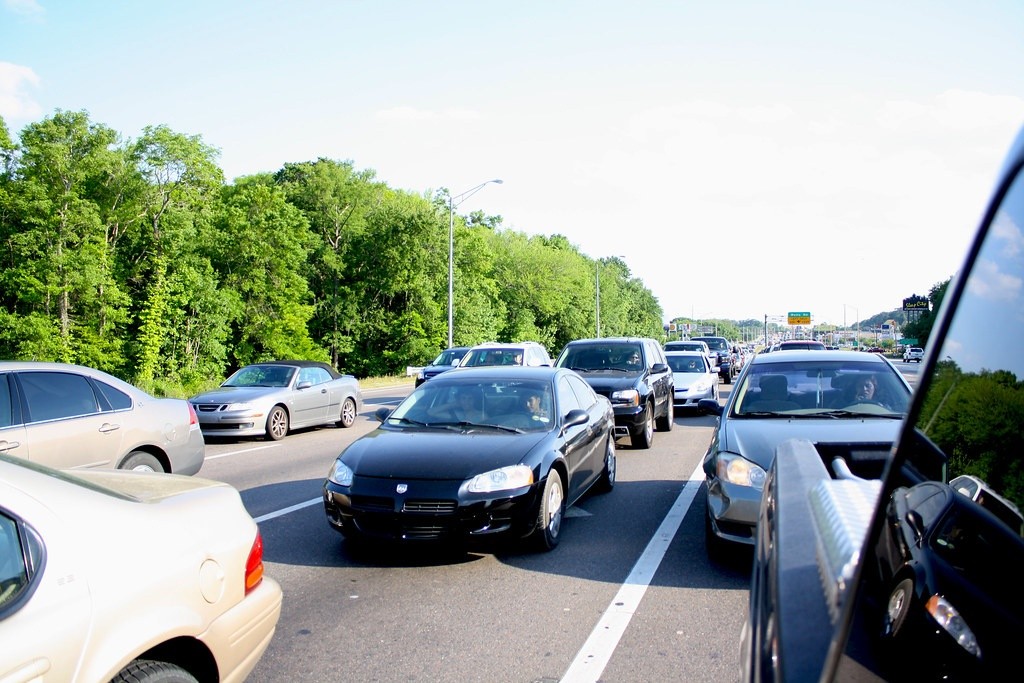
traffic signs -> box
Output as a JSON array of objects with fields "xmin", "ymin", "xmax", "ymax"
[{"xmin": 788, "ymin": 312, "xmax": 810, "ymax": 326}]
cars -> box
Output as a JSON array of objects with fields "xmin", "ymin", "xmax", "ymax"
[
  {"xmin": 902, "ymin": 348, "xmax": 924, "ymax": 363},
  {"xmin": 190, "ymin": 361, "xmax": 359, "ymax": 440},
  {"xmin": 0, "ymin": 357, "xmax": 205, "ymax": 475},
  {"xmin": 325, "ymin": 365, "xmax": 615, "ymax": 558},
  {"xmin": 418, "ymin": 343, "xmax": 551, "ymax": 388},
  {"xmin": 0, "ymin": 461, "xmax": 285, "ymax": 683},
  {"xmin": 557, "ymin": 335, "xmax": 760, "ymax": 448},
  {"xmin": 700, "ymin": 340, "xmax": 919, "ymax": 567}
]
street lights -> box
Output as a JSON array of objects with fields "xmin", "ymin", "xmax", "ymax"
[
  {"xmin": 447, "ymin": 178, "xmax": 503, "ymax": 349},
  {"xmin": 594, "ymin": 255, "xmax": 626, "ymax": 338}
]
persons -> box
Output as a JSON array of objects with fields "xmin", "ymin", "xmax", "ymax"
[
  {"xmin": 426, "ymin": 388, "xmax": 489, "ymax": 424},
  {"xmin": 688, "ymin": 360, "xmax": 699, "ymax": 371},
  {"xmin": 867, "ymin": 347, "xmax": 885, "ymax": 352},
  {"xmin": 630, "ymin": 351, "xmax": 639, "ymax": 364},
  {"xmin": 521, "ymin": 391, "xmax": 544, "ymax": 412},
  {"xmin": 503, "ymin": 352, "xmax": 518, "ymax": 365},
  {"xmin": 845, "ymin": 374, "xmax": 884, "ymax": 402}
]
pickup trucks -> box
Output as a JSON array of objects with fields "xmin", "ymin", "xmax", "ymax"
[{"xmin": 748, "ymin": 118, "xmax": 1024, "ymax": 683}]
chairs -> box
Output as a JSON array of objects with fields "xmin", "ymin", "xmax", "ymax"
[
  {"xmin": 750, "ymin": 375, "xmax": 801, "ymax": 412},
  {"xmin": 827, "ymin": 375, "xmax": 890, "ymax": 409}
]
